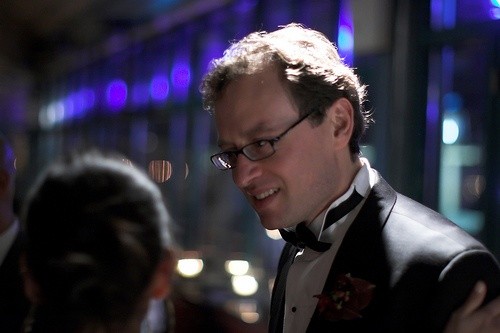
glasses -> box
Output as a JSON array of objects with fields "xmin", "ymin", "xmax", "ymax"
[{"xmin": 210, "ymin": 107, "xmax": 318, "ymax": 171}]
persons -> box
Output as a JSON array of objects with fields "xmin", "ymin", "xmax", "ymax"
[
  {"xmin": 0, "ymin": 147, "xmax": 500, "ymax": 333},
  {"xmin": 198, "ymin": 23, "xmax": 500, "ymax": 333}
]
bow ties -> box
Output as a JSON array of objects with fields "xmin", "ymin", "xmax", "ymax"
[{"xmin": 278, "ymin": 189, "xmax": 364, "ymax": 253}]
